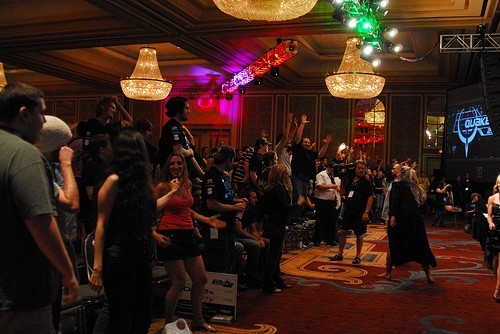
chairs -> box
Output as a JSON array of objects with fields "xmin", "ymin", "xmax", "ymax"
[
  {"xmin": 432, "ymin": 189, "xmax": 458, "ymax": 229},
  {"xmin": 285, "ymin": 217, "xmax": 317, "ymax": 252},
  {"xmin": 53, "ymin": 232, "xmax": 189, "ymax": 334}
]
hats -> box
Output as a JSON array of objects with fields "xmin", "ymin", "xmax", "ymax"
[
  {"xmin": 34, "ymin": 115, "xmax": 73, "ymax": 153},
  {"xmin": 212, "ymin": 145, "xmax": 236, "ymax": 161},
  {"xmin": 255, "ymin": 137, "xmax": 273, "ymax": 146}
]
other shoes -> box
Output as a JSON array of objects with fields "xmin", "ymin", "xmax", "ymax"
[
  {"xmin": 251, "ymin": 282, "xmax": 263, "ymax": 288},
  {"xmin": 278, "ymin": 284, "xmax": 292, "ymax": 289},
  {"xmin": 280, "ymin": 276, "xmax": 285, "ymax": 283},
  {"xmin": 431, "ymin": 223, "xmax": 445, "ymax": 228},
  {"xmin": 326, "ymin": 241, "xmax": 338, "ymax": 246},
  {"xmin": 263, "ymin": 288, "xmax": 282, "ymax": 293},
  {"xmin": 197, "ymin": 319, "xmax": 217, "ymax": 333},
  {"xmin": 331, "ymin": 255, "xmax": 343, "ymax": 261},
  {"xmin": 493, "ymin": 292, "xmax": 500, "ymax": 303},
  {"xmin": 352, "ymin": 257, "xmax": 361, "ymax": 264},
  {"xmin": 239, "ymin": 283, "xmax": 248, "ymax": 289}
]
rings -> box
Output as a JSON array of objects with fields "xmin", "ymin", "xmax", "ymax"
[{"xmin": 91, "ymin": 285, "xmax": 93, "ymax": 287}]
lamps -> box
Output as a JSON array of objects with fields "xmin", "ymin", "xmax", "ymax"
[
  {"xmin": 239, "ymin": 84, "xmax": 247, "ymax": 93},
  {"xmin": 213, "ymin": 0, "xmax": 318, "ymax": 22},
  {"xmin": 324, "ymin": 38, "xmax": 386, "ymax": 100},
  {"xmin": 271, "ymin": 67, "xmax": 279, "ymax": 76},
  {"xmin": 120, "ymin": 43, "xmax": 172, "ymax": 101},
  {"xmin": 225, "ymin": 92, "xmax": 233, "ymax": 100},
  {"xmin": 254, "ymin": 76, "xmax": 263, "ymax": 85}
]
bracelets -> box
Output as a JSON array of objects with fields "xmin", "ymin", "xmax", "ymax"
[{"xmin": 366, "ymin": 210, "xmax": 369, "ymax": 213}]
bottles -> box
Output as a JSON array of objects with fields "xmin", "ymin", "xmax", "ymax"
[{"xmin": 234, "ymin": 201, "xmax": 246, "ymax": 223}]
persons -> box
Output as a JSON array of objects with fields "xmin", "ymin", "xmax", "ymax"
[
  {"xmin": 35, "ymin": 96, "xmax": 500, "ymax": 334},
  {"xmin": 0, "ymin": 83, "xmax": 80, "ymax": 334}
]
